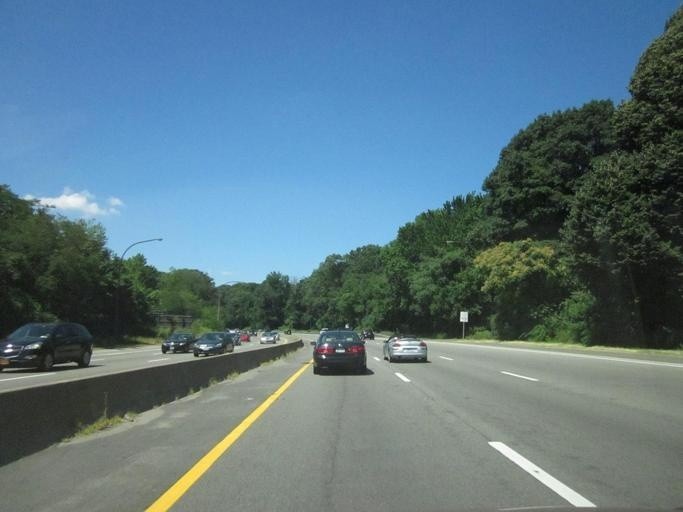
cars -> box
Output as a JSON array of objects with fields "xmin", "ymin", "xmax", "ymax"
[
  {"xmin": 229, "ymin": 330, "xmax": 250, "ymax": 345},
  {"xmin": 311, "ymin": 329, "xmax": 366, "ymax": 374},
  {"xmin": 361, "ymin": 328, "xmax": 373, "ymax": 340},
  {"xmin": 162, "ymin": 331, "xmax": 198, "ymax": 353},
  {"xmin": 0, "ymin": 321, "xmax": 94, "ymax": 371},
  {"xmin": 382, "ymin": 333, "xmax": 427, "ymax": 363},
  {"xmin": 194, "ymin": 331, "xmax": 234, "ymax": 356},
  {"xmin": 260, "ymin": 330, "xmax": 279, "ymax": 344}
]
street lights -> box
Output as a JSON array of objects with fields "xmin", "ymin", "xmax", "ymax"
[{"xmin": 115, "ymin": 238, "xmax": 163, "ymax": 340}]
